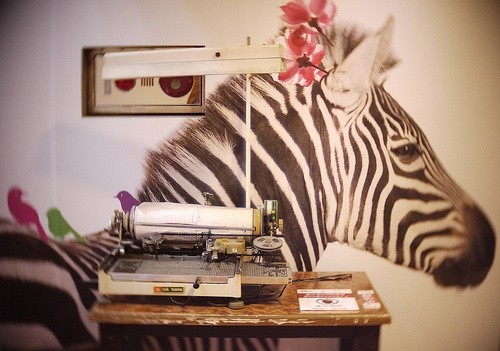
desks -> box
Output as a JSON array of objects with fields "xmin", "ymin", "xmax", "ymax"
[{"xmin": 87, "ymin": 269, "xmax": 392, "ymax": 351}]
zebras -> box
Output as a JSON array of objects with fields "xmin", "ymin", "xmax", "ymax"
[{"xmin": 0, "ymin": 18, "xmax": 496, "ymax": 351}]
